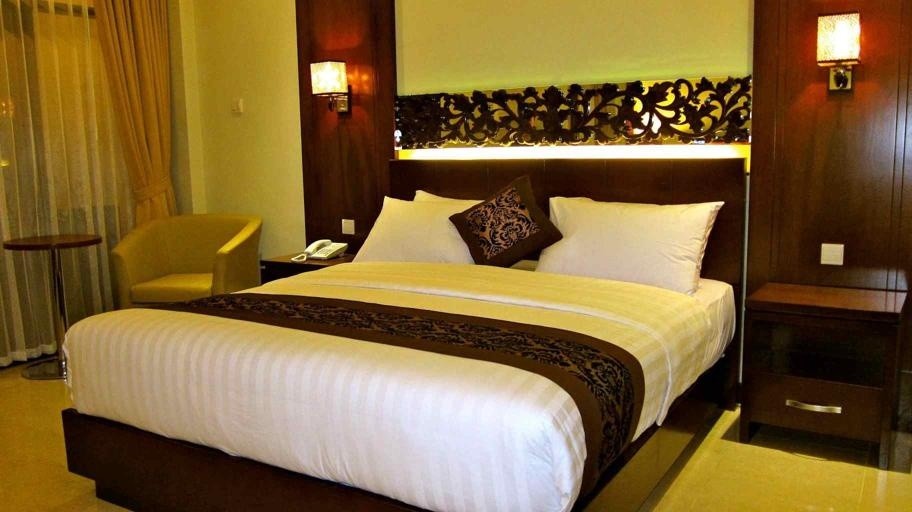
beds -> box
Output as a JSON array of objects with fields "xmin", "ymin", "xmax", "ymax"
[{"xmin": 61, "ymin": 158, "xmax": 754, "ymax": 512}]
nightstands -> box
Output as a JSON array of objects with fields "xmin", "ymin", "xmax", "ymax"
[
  {"xmin": 260, "ymin": 253, "xmax": 355, "ymax": 284},
  {"xmin": 739, "ymin": 282, "xmax": 908, "ymax": 470}
]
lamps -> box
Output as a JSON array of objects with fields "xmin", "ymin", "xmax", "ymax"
[
  {"xmin": 310, "ymin": 61, "xmax": 349, "ymax": 112},
  {"xmin": 817, "ymin": 13, "xmax": 860, "ymax": 92}
]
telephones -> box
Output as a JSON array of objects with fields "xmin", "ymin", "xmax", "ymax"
[{"xmin": 304, "ymin": 239, "xmax": 349, "ymax": 260}]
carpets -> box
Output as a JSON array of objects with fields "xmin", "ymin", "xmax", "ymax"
[{"xmin": 639, "ymin": 403, "xmax": 912, "ymax": 512}]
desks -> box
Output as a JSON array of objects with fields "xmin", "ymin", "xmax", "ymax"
[{"xmin": 3, "ymin": 234, "xmax": 102, "ymax": 380}]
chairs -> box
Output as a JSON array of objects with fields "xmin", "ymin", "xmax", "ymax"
[{"xmin": 109, "ymin": 214, "xmax": 263, "ymax": 309}]
